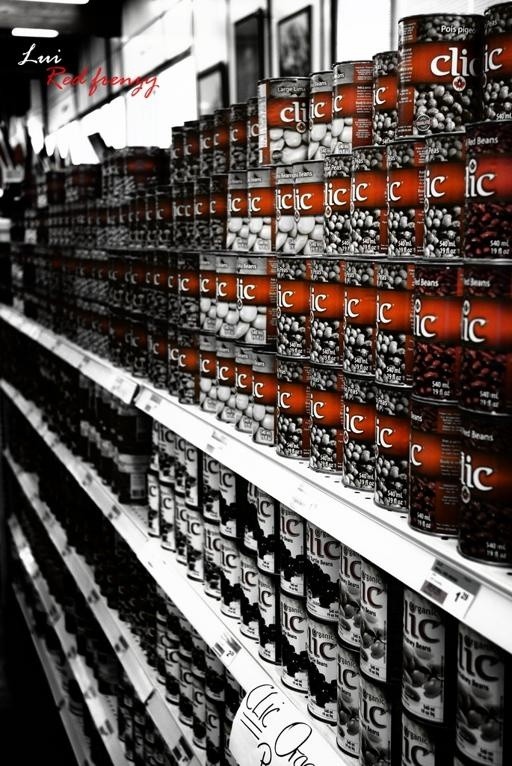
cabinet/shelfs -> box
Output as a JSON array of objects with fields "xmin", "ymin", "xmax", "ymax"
[{"xmin": 0, "ymin": 297, "xmax": 512, "ymax": 760}]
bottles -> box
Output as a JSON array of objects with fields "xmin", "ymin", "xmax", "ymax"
[{"xmin": 30, "ymin": 354, "xmax": 145, "ymax": 504}]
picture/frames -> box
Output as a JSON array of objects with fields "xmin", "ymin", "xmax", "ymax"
[
  {"xmin": 276, "ymin": 4, "xmax": 313, "ymax": 77},
  {"xmin": 232, "ymin": 7, "xmax": 266, "ymax": 103},
  {"xmin": 196, "ymin": 61, "xmax": 228, "ymax": 119}
]
